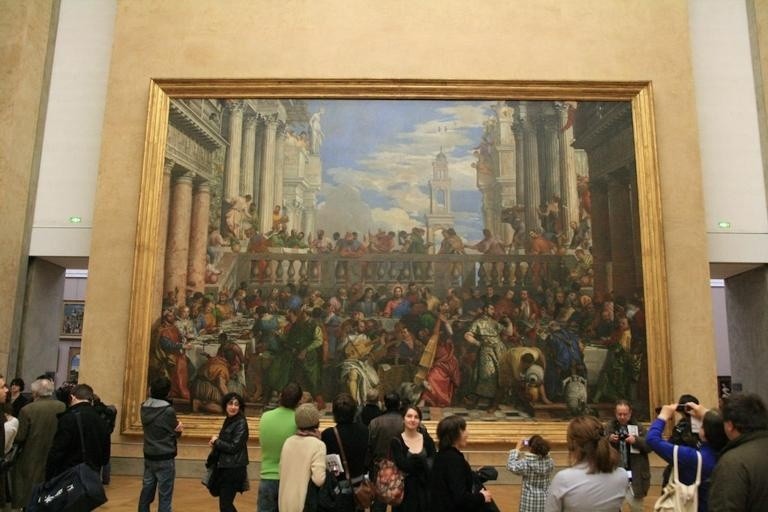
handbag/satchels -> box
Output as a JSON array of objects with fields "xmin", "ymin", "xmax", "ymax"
[
  {"xmin": 23, "ymin": 459, "xmax": 109, "ymax": 511},
  {"xmin": 651, "ymin": 442, "xmax": 704, "ymax": 512},
  {"xmin": 473, "ymin": 478, "xmax": 502, "ymax": 512},
  {"xmin": 352, "ymin": 477, "xmax": 376, "ymax": 510},
  {"xmin": 200, "ymin": 463, "xmax": 216, "ymax": 487},
  {"xmin": 373, "ymin": 444, "xmax": 407, "ymax": 508}
]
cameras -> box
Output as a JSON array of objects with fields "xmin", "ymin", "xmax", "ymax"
[
  {"xmin": 523, "ymin": 440, "xmax": 529, "ymax": 445},
  {"xmin": 618, "ymin": 433, "xmax": 628, "ymax": 441},
  {"xmin": 676, "ymin": 405, "xmax": 690, "ymax": 411}
]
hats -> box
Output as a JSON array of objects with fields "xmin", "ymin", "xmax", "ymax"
[{"xmin": 294, "ymin": 402, "xmax": 321, "ymax": 428}]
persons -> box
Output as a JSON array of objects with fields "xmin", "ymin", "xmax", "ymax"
[
  {"xmin": 207, "ymin": 393, "xmax": 249, "ymax": 511},
  {"xmin": 545, "ymin": 418, "xmax": 629, "ymax": 512},
  {"xmin": 138, "ymin": 377, "xmax": 182, "ymax": 512},
  {"xmin": 605, "ymin": 400, "xmax": 653, "ymax": 511},
  {"xmin": 645, "ymin": 394, "xmax": 768, "ymax": 512},
  {"xmin": 0, "ymin": 375, "xmax": 117, "ymax": 512},
  {"xmin": 506, "ymin": 435, "xmax": 553, "ymax": 512},
  {"xmin": 257, "ymin": 381, "xmax": 492, "ymax": 512}
]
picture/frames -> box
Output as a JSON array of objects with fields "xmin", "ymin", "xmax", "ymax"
[{"xmin": 120, "ymin": 76, "xmax": 676, "ymax": 446}]
